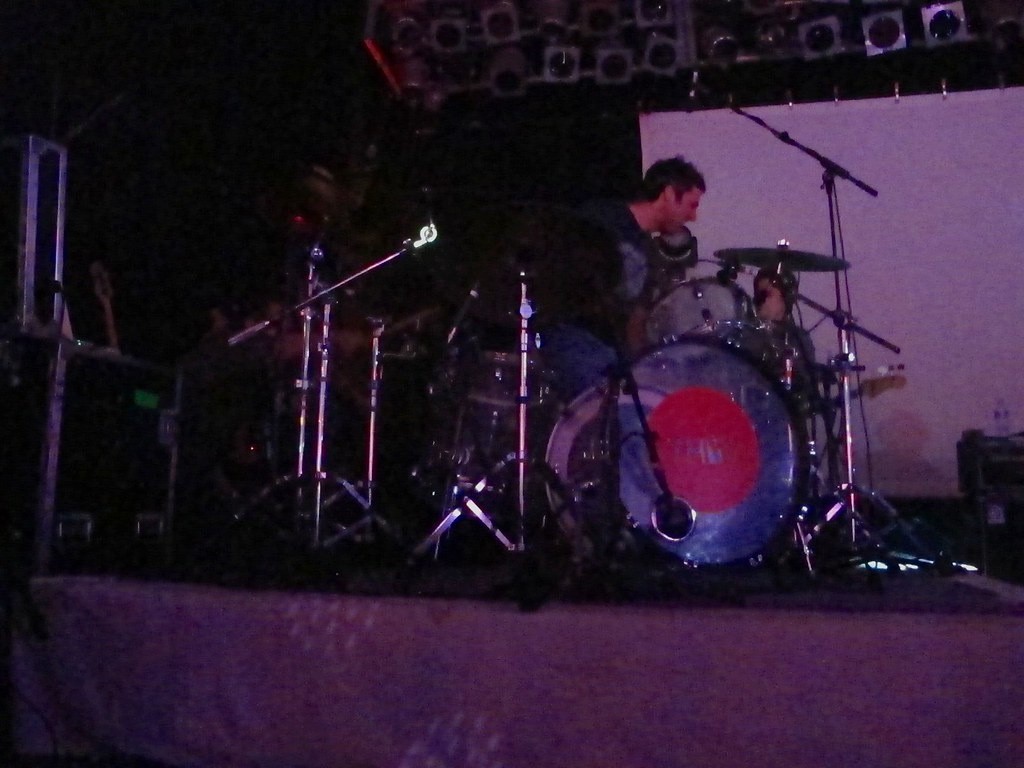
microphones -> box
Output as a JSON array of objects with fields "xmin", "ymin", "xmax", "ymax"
[
  {"xmin": 447, "ymin": 283, "xmax": 481, "ymax": 343},
  {"xmin": 684, "ymin": 64, "xmax": 700, "ymax": 113},
  {"xmin": 782, "ymin": 272, "xmax": 795, "ymax": 314},
  {"xmin": 661, "ymin": 489, "xmax": 689, "ymax": 532}
]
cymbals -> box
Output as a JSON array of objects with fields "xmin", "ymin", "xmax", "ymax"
[
  {"xmin": 439, "ymin": 191, "xmax": 622, "ymax": 327},
  {"xmin": 712, "ymin": 235, "xmax": 854, "ymax": 277}
]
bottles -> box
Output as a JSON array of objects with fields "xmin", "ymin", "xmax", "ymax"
[{"xmin": 994, "ymin": 398, "xmax": 1009, "ymax": 437}]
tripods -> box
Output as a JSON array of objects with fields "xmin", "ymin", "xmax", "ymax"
[
  {"xmin": 187, "ymin": 216, "xmax": 631, "ymax": 616},
  {"xmin": 696, "ymin": 82, "xmax": 954, "ymax": 577}
]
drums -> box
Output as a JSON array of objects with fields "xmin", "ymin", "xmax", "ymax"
[
  {"xmin": 642, "ymin": 270, "xmax": 782, "ymax": 379},
  {"xmin": 416, "ymin": 348, "xmax": 560, "ymax": 527},
  {"xmin": 545, "ymin": 325, "xmax": 811, "ymax": 577}
]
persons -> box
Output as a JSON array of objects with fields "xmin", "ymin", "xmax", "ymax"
[
  {"xmin": 536, "ymin": 152, "xmax": 707, "ymax": 402},
  {"xmin": 744, "ymin": 259, "xmax": 816, "ymax": 387}
]
